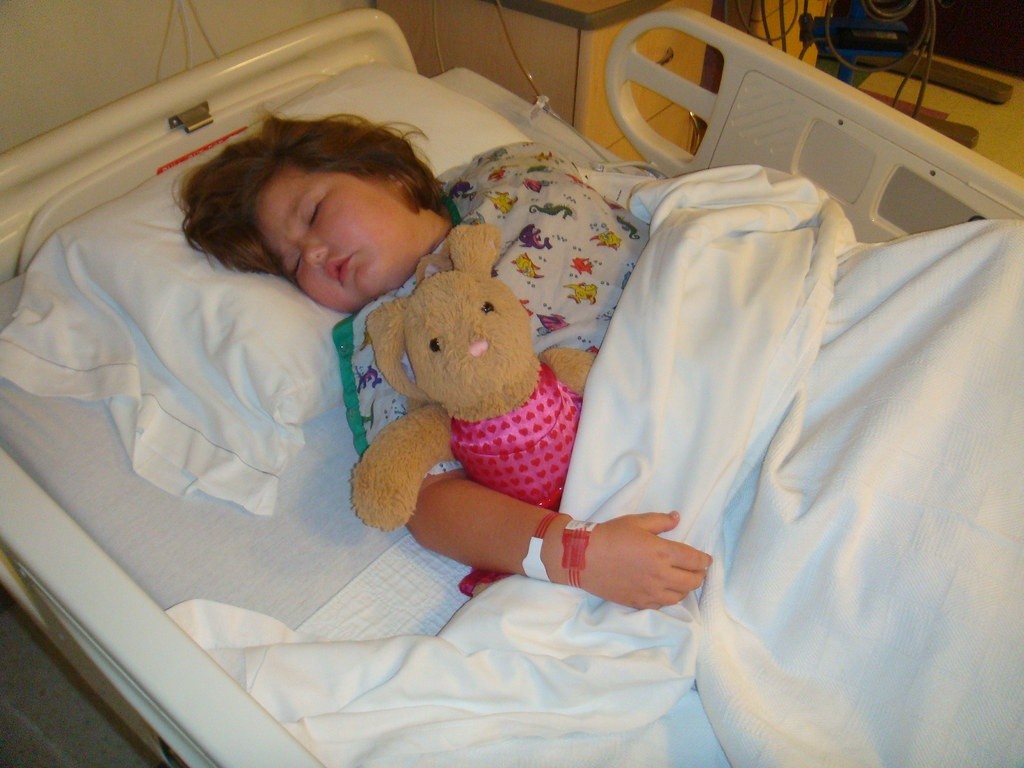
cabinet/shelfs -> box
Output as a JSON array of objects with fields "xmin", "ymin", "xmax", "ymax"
[{"xmin": 370, "ymin": 0, "xmax": 830, "ymax": 175}]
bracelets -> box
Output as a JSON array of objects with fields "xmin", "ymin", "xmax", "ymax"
[{"xmin": 520, "ymin": 509, "xmax": 601, "ymax": 590}]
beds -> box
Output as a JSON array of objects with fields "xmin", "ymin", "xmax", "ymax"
[{"xmin": 0, "ymin": 8, "xmax": 1024, "ymax": 768}]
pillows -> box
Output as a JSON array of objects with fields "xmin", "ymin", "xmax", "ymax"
[{"xmin": 0, "ymin": 58, "xmax": 534, "ymax": 521}]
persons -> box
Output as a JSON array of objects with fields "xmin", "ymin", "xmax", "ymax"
[{"xmin": 179, "ymin": 109, "xmax": 714, "ymax": 611}]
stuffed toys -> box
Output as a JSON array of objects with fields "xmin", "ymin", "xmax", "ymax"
[{"xmin": 347, "ymin": 221, "xmax": 602, "ymax": 533}]
